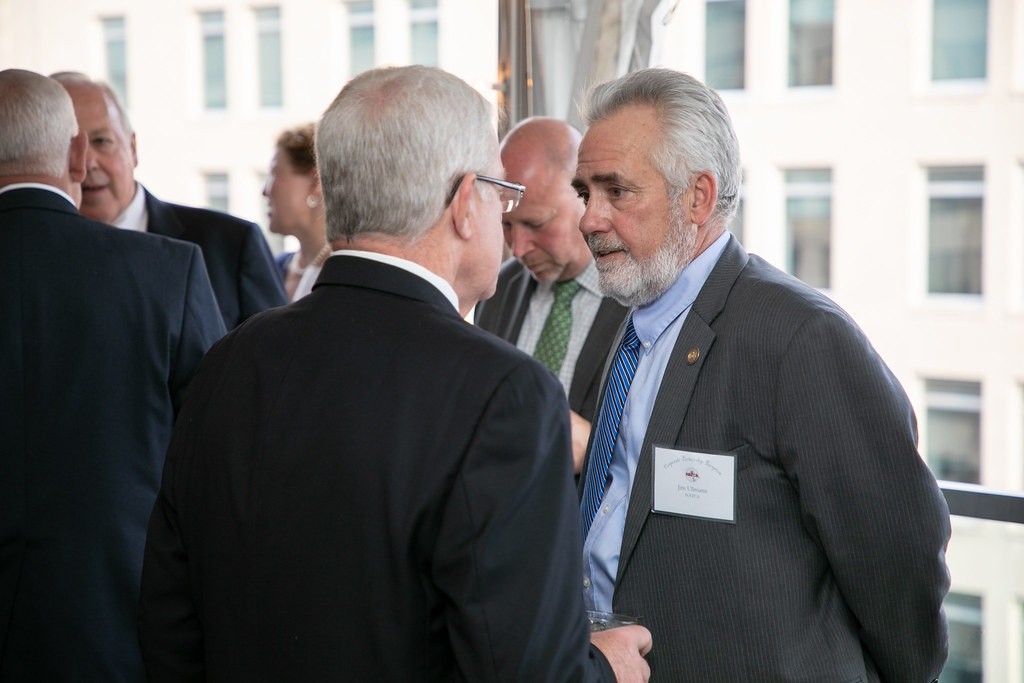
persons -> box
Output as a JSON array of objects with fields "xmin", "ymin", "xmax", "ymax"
[
  {"xmin": 140, "ymin": 65, "xmax": 653, "ymax": 683},
  {"xmin": 575, "ymin": 69, "xmax": 950, "ymax": 683},
  {"xmin": 0, "ymin": 69, "xmax": 226, "ymax": 683},
  {"xmin": 47, "ymin": 72, "xmax": 289, "ymax": 331},
  {"xmin": 472, "ymin": 117, "xmax": 629, "ymax": 476},
  {"xmin": 263, "ymin": 122, "xmax": 332, "ymax": 304}
]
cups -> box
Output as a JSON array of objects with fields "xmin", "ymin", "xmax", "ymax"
[{"xmin": 587, "ymin": 609, "xmax": 638, "ymax": 631}]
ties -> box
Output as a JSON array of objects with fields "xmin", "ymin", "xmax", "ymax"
[
  {"xmin": 581, "ymin": 312, "xmax": 641, "ymax": 552},
  {"xmin": 532, "ymin": 280, "xmax": 581, "ymax": 376}
]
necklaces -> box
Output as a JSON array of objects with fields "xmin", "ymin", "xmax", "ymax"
[{"xmin": 290, "ymin": 243, "xmax": 330, "ymax": 275}]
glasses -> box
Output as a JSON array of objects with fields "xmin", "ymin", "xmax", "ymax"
[{"xmin": 445, "ymin": 173, "xmax": 526, "ymax": 212}]
